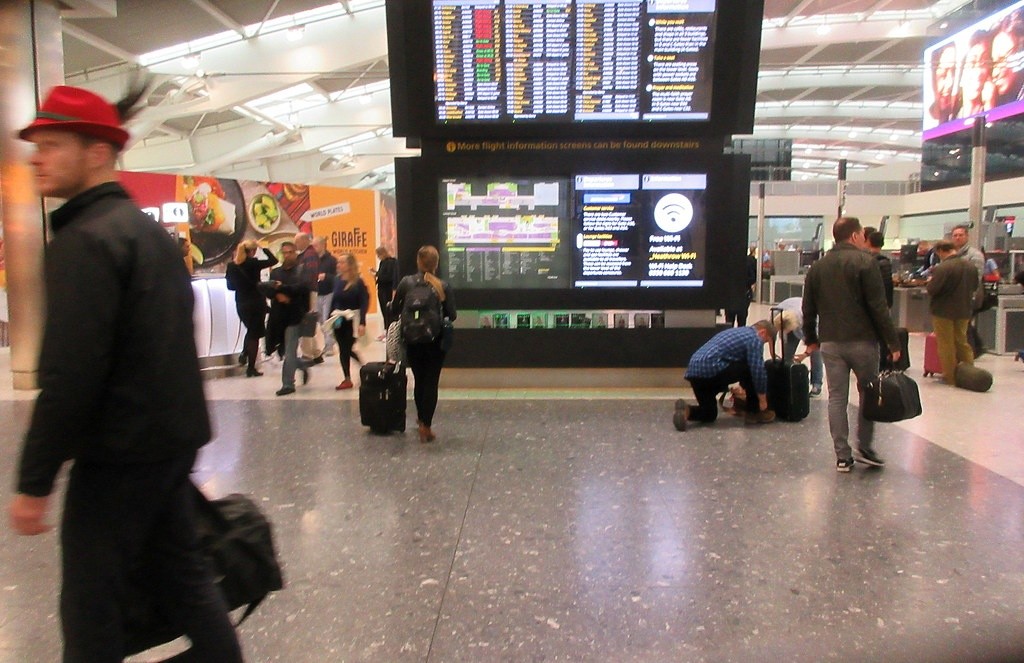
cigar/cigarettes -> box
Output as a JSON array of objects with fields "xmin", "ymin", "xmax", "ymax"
[{"xmin": 317, "ymin": 278, "xmax": 320, "ymax": 282}]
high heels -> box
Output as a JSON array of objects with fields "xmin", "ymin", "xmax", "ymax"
[{"xmin": 419, "ymin": 423, "xmax": 435, "ymax": 443}]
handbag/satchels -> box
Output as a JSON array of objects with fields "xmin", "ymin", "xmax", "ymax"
[
  {"xmin": 977, "ymin": 275, "xmax": 1000, "ymax": 310},
  {"xmin": 864, "ymin": 354, "xmax": 922, "ymax": 422},
  {"xmin": 101, "ymin": 480, "xmax": 283, "ymax": 658},
  {"xmin": 720, "ymin": 388, "xmax": 747, "ymax": 415},
  {"xmin": 296, "ymin": 322, "xmax": 325, "ymax": 362}
]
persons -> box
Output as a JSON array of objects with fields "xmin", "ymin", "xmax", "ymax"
[
  {"xmin": 863, "ymin": 227, "xmax": 894, "ymax": 373},
  {"xmin": 673, "ymin": 320, "xmax": 776, "ymax": 431},
  {"xmin": 801, "ymin": 216, "xmax": 900, "ymax": 474},
  {"xmin": 310, "ymin": 237, "xmax": 336, "ymax": 358},
  {"xmin": 294, "ymin": 232, "xmax": 321, "ymax": 360},
  {"xmin": 226, "ymin": 239, "xmax": 280, "ymax": 377},
  {"xmin": 13, "ymin": 88, "xmax": 252, "ymax": 663},
  {"xmin": 178, "ymin": 238, "xmax": 197, "ymax": 279},
  {"xmin": 916, "ymin": 225, "xmax": 1000, "ymax": 385},
  {"xmin": 328, "ymin": 252, "xmax": 368, "ymax": 389},
  {"xmin": 768, "ymin": 296, "xmax": 824, "ymax": 397},
  {"xmin": 386, "ymin": 245, "xmax": 457, "ymax": 444},
  {"xmin": 370, "ymin": 246, "xmax": 399, "ymax": 342},
  {"xmin": 265, "ymin": 240, "xmax": 310, "ymax": 397},
  {"xmin": 928, "ymin": 6, "xmax": 1024, "ymax": 124}
]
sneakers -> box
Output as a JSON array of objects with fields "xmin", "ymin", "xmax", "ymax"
[
  {"xmin": 745, "ymin": 410, "xmax": 776, "ymax": 424},
  {"xmin": 673, "ymin": 399, "xmax": 689, "ymax": 430},
  {"xmin": 856, "ymin": 449, "xmax": 885, "ymax": 466},
  {"xmin": 837, "ymin": 458, "xmax": 855, "ymax": 472}
]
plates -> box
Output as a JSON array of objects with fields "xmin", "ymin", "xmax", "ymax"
[
  {"xmin": 190, "ymin": 179, "xmax": 245, "ymax": 267},
  {"xmin": 256, "ymin": 230, "xmax": 311, "ymax": 270}
]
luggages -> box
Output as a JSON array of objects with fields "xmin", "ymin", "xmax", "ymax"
[
  {"xmin": 894, "ymin": 328, "xmax": 911, "ymax": 371},
  {"xmin": 764, "ymin": 308, "xmax": 810, "ymax": 421},
  {"xmin": 923, "ymin": 329, "xmax": 942, "ymax": 378},
  {"xmin": 359, "ymin": 312, "xmax": 407, "ymax": 431}
]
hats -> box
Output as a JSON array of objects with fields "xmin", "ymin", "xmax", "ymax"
[{"xmin": 19, "ymin": 86, "xmax": 130, "ymax": 150}]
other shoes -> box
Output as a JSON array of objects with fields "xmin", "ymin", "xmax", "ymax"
[
  {"xmin": 247, "ymin": 366, "xmax": 263, "ymax": 377},
  {"xmin": 809, "ymin": 387, "xmax": 822, "ymax": 397},
  {"xmin": 326, "ymin": 347, "xmax": 334, "ymax": 357},
  {"xmin": 276, "ymin": 387, "xmax": 295, "ymax": 395},
  {"xmin": 375, "ymin": 333, "xmax": 386, "ymax": 342},
  {"xmin": 336, "ymin": 380, "xmax": 353, "ymax": 389},
  {"xmin": 239, "ymin": 354, "xmax": 247, "ymax": 365},
  {"xmin": 303, "ymin": 369, "xmax": 309, "ymax": 385}
]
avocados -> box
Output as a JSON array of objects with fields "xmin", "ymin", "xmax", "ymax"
[{"xmin": 254, "ymin": 196, "xmax": 278, "ymax": 230}]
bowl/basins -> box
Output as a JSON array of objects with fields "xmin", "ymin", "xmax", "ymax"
[{"xmin": 248, "ymin": 193, "xmax": 280, "ymax": 234}]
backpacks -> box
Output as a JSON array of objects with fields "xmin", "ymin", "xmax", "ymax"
[{"xmin": 402, "ymin": 276, "xmax": 443, "ymax": 344}]
